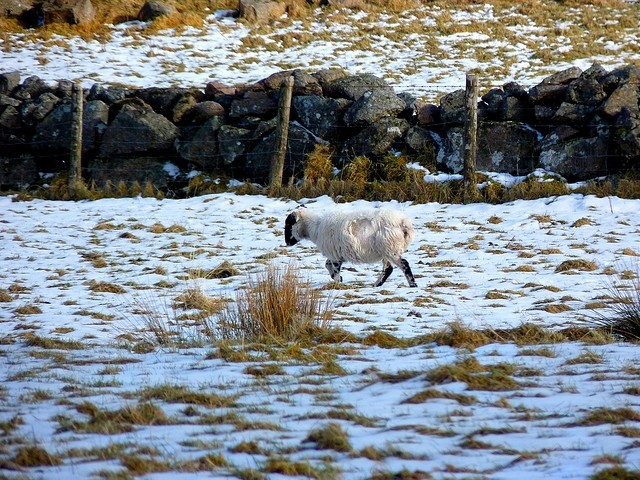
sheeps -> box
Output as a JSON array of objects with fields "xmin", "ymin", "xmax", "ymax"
[{"xmin": 285, "ymin": 204, "xmax": 418, "ymax": 288}]
